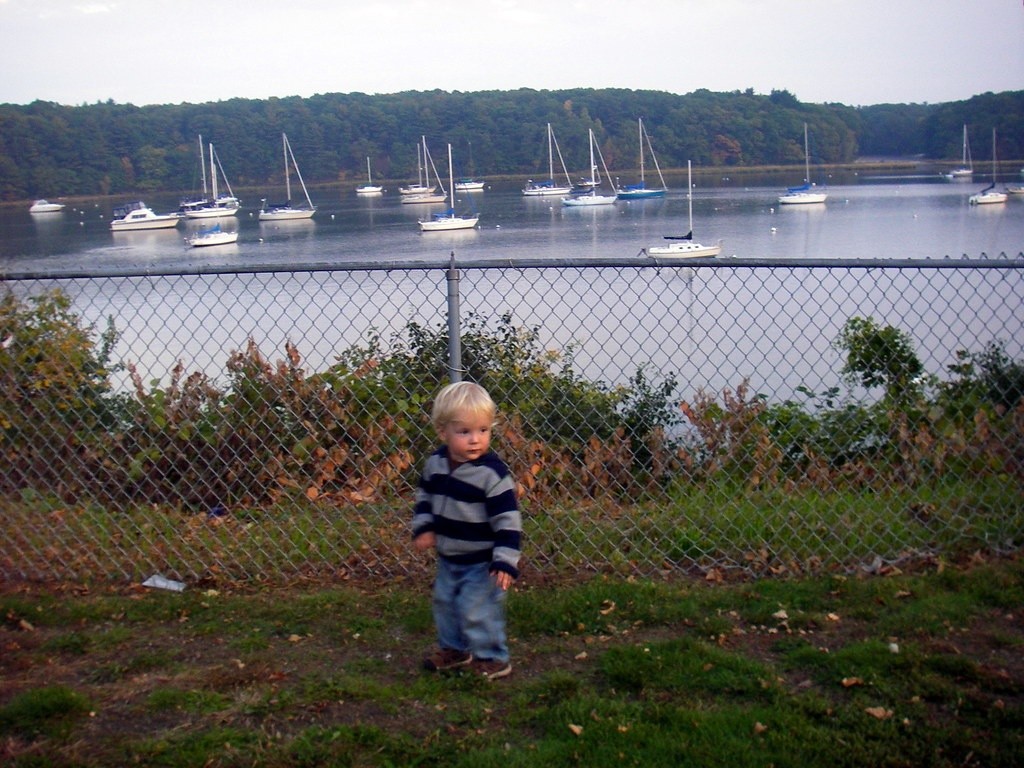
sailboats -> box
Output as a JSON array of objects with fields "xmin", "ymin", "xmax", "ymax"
[
  {"xmin": 521, "ymin": 123, "xmax": 573, "ymax": 195},
  {"xmin": 185, "ymin": 142, "xmax": 240, "ymax": 217},
  {"xmin": 648, "ymin": 160, "xmax": 721, "ymax": 258},
  {"xmin": 257, "ymin": 132, "xmax": 316, "ymax": 220},
  {"xmin": 400, "ymin": 135, "xmax": 448, "ymax": 204},
  {"xmin": 948, "ymin": 124, "xmax": 974, "ymax": 177},
  {"xmin": 355, "ymin": 156, "xmax": 383, "ymax": 192},
  {"xmin": 777, "ymin": 122, "xmax": 828, "ymax": 203},
  {"xmin": 417, "ymin": 143, "xmax": 479, "ymax": 230},
  {"xmin": 398, "ymin": 143, "xmax": 437, "ymax": 193},
  {"xmin": 576, "ymin": 153, "xmax": 602, "ymax": 187},
  {"xmin": 617, "ymin": 118, "xmax": 668, "ymax": 200},
  {"xmin": 561, "ymin": 128, "xmax": 619, "ymax": 206},
  {"xmin": 969, "ymin": 128, "xmax": 1008, "ymax": 205},
  {"xmin": 180, "ymin": 135, "xmax": 213, "ymax": 210}
]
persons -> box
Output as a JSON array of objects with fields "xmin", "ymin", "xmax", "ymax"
[{"xmin": 410, "ymin": 381, "xmax": 523, "ymax": 683}]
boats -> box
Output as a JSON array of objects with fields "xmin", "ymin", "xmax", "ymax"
[
  {"xmin": 188, "ymin": 230, "xmax": 239, "ymax": 247},
  {"xmin": 1004, "ymin": 186, "xmax": 1024, "ymax": 194},
  {"xmin": 29, "ymin": 199, "xmax": 185, "ymax": 231},
  {"xmin": 458, "ymin": 178, "xmax": 474, "ymax": 182},
  {"xmin": 454, "ymin": 182, "xmax": 484, "ymax": 189}
]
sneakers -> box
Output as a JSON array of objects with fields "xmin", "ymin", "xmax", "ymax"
[
  {"xmin": 457, "ymin": 657, "xmax": 513, "ymax": 681},
  {"xmin": 423, "ymin": 646, "xmax": 474, "ymax": 672}
]
